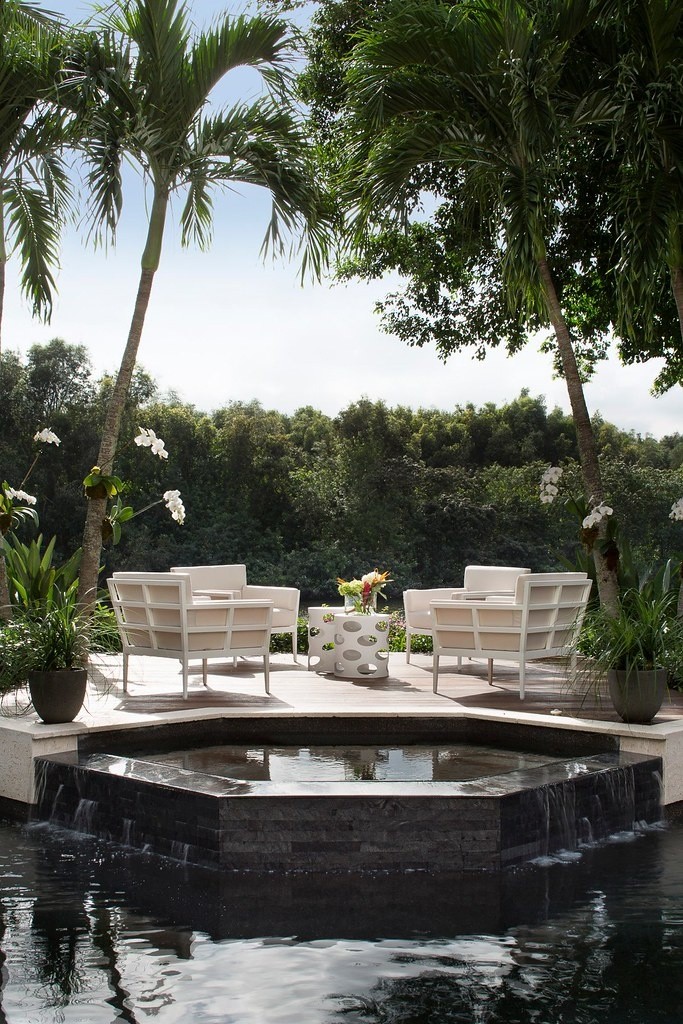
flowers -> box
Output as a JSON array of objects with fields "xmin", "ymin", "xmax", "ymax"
[{"xmin": 336, "ymin": 568, "xmax": 393, "ymax": 615}]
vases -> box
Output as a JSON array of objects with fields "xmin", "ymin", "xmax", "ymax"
[{"xmin": 344, "ymin": 592, "xmax": 377, "ymax": 616}]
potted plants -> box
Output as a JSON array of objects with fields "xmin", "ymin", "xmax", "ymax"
[
  {"xmin": 0, "ymin": 584, "xmax": 150, "ymax": 722},
  {"xmin": 555, "ymin": 580, "xmax": 683, "ymax": 723}
]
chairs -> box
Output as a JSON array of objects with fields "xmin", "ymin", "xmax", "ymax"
[
  {"xmin": 106, "ymin": 571, "xmax": 274, "ymax": 700},
  {"xmin": 402, "ymin": 565, "xmax": 531, "ymax": 670},
  {"xmin": 169, "ymin": 563, "xmax": 300, "ymax": 675},
  {"xmin": 429, "ymin": 572, "xmax": 592, "ymax": 700}
]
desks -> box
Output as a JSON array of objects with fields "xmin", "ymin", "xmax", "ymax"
[
  {"xmin": 307, "ymin": 606, "xmax": 345, "ymax": 670},
  {"xmin": 333, "ymin": 613, "xmax": 388, "ymax": 678}
]
